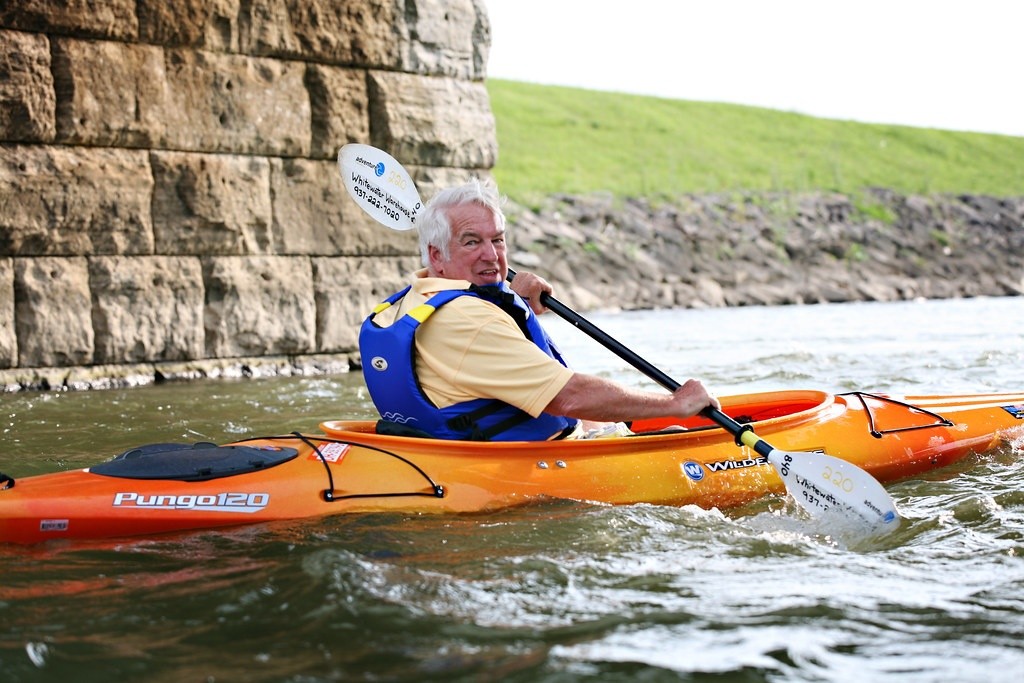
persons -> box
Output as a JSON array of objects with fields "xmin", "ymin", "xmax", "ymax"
[{"xmin": 361, "ymin": 182, "xmax": 718, "ymax": 442}]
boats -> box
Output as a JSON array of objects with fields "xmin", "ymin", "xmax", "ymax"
[{"xmin": 0, "ymin": 388, "xmax": 1024, "ymax": 549}]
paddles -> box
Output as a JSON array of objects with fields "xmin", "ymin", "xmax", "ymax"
[{"xmin": 338, "ymin": 144, "xmax": 903, "ymax": 544}]
